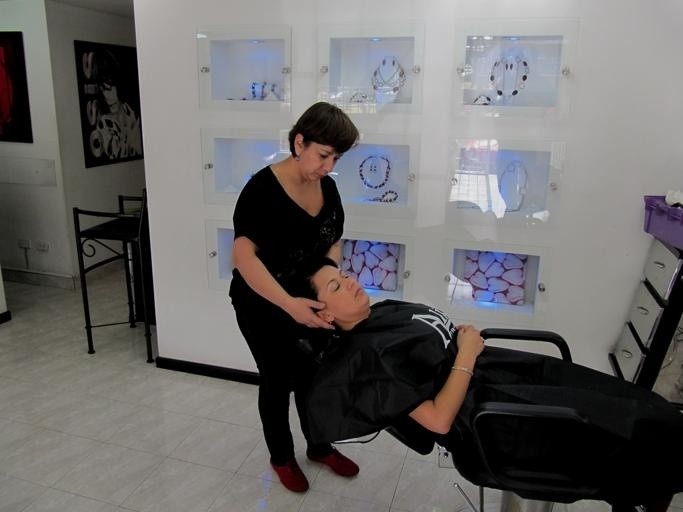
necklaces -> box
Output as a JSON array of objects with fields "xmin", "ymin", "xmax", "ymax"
[
  {"xmin": 490, "ymin": 57, "xmax": 530, "ymax": 96},
  {"xmin": 359, "ymin": 156, "xmax": 391, "ymax": 189},
  {"xmin": 371, "ymin": 63, "xmax": 406, "ymax": 94}
]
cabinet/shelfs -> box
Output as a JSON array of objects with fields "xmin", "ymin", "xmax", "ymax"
[{"xmin": 607, "ymin": 236, "xmax": 682, "ymax": 391}]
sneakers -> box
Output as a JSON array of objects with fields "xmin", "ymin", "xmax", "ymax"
[
  {"xmin": 271, "ymin": 457, "xmax": 309, "ymax": 492},
  {"xmin": 307, "ymin": 448, "xmax": 359, "ymax": 476}
]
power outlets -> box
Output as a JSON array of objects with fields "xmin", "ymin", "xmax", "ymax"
[
  {"xmin": 36, "ymin": 240, "xmax": 51, "ymax": 251},
  {"xmin": 18, "ymin": 239, "xmax": 32, "ymax": 249}
]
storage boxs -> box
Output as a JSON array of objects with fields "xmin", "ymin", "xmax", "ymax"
[{"xmin": 643, "ymin": 195, "xmax": 683, "ymax": 250}]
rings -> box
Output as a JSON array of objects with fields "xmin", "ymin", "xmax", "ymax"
[{"xmin": 481, "ymin": 336, "xmax": 484, "ymax": 344}]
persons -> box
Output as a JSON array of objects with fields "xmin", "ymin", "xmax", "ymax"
[
  {"xmin": 296, "ymin": 257, "xmax": 683, "ymax": 504},
  {"xmin": 229, "ymin": 103, "xmax": 359, "ymax": 493}
]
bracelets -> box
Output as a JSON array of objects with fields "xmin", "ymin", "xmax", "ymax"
[
  {"xmin": 252, "ymin": 81, "xmax": 257, "ymax": 98},
  {"xmin": 260, "ymin": 82, "xmax": 266, "ymax": 98},
  {"xmin": 349, "ymin": 92, "xmax": 366, "ymax": 103},
  {"xmin": 271, "ymin": 84, "xmax": 286, "ymax": 101},
  {"xmin": 472, "ymin": 94, "xmax": 490, "ymax": 105},
  {"xmin": 452, "ymin": 366, "xmax": 474, "ymax": 375},
  {"xmin": 379, "ymin": 190, "xmax": 399, "ymax": 202}
]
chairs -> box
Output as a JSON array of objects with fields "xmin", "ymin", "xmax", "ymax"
[
  {"xmin": 284, "ymin": 328, "xmax": 592, "ymax": 511},
  {"xmin": 72, "ymin": 187, "xmax": 156, "ymax": 363}
]
